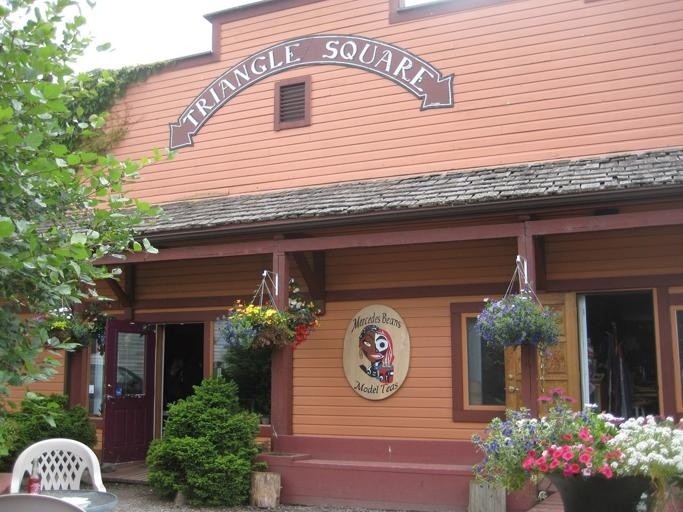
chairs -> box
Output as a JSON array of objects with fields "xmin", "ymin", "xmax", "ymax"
[{"xmin": 1, "ymin": 436, "xmax": 107, "ymax": 512}]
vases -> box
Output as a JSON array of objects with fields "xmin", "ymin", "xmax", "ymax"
[{"xmin": 539, "ymin": 468, "xmax": 651, "ymax": 512}]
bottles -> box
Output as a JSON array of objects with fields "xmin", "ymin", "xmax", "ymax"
[{"xmin": 28, "ymin": 457, "xmax": 41, "ymax": 496}]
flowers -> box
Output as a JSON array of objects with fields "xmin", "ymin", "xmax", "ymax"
[
  {"xmin": 218, "ymin": 299, "xmax": 294, "ymax": 353},
  {"xmin": 472, "ymin": 287, "xmax": 567, "ymax": 358},
  {"xmin": 471, "ymin": 385, "xmax": 683, "ymax": 512}
]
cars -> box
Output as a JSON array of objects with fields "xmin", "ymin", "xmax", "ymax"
[{"xmin": 89, "ymin": 364, "xmax": 142, "ymax": 415}]
